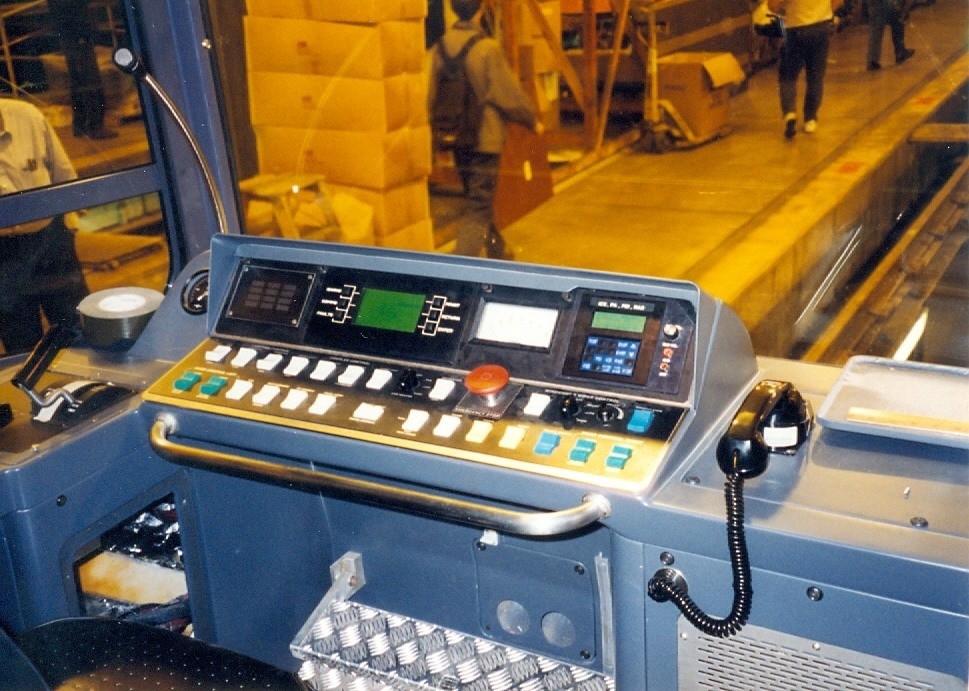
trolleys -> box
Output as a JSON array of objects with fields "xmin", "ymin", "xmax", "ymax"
[{"xmin": 632, "ymin": 20, "xmax": 733, "ymax": 152}]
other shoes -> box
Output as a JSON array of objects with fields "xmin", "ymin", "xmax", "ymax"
[
  {"xmin": 487, "ymin": 248, "xmax": 515, "ymax": 260},
  {"xmin": 867, "ymin": 61, "xmax": 882, "ymax": 70},
  {"xmin": 89, "ymin": 128, "xmax": 119, "ymax": 139},
  {"xmin": 803, "ymin": 119, "xmax": 816, "ymax": 133},
  {"xmin": 895, "ymin": 48, "xmax": 915, "ymax": 62},
  {"xmin": 784, "ymin": 113, "xmax": 797, "ymax": 138}
]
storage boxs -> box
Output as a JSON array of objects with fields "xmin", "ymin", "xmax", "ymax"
[{"xmin": 658, "ymin": 51, "xmax": 746, "ymax": 138}]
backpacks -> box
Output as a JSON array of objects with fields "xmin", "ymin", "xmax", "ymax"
[{"xmin": 428, "ymin": 31, "xmax": 487, "ymax": 152}]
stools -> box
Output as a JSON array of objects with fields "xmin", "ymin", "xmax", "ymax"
[{"xmin": 237, "ymin": 173, "xmax": 342, "ymax": 244}]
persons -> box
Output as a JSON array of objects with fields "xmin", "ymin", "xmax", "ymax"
[
  {"xmin": 48, "ymin": 0, "xmax": 122, "ymax": 140},
  {"xmin": 428, "ymin": 0, "xmax": 547, "ymax": 262},
  {"xmin": 0, "ymin": 98, "xmax": 94, "ymax": 353},
  {"xmin": 769, "ymin": 0, "xmax": 916, "ymax": 139}
]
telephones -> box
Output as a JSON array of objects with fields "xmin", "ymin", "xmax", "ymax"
[{"xmin": 716, "ymin": 379, "xmax": 814, "ymax": 480}]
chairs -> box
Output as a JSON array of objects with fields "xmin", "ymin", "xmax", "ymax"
[{"xmin": 0, "ymin": 617, "xmax": 315, "ymax": 691}]
